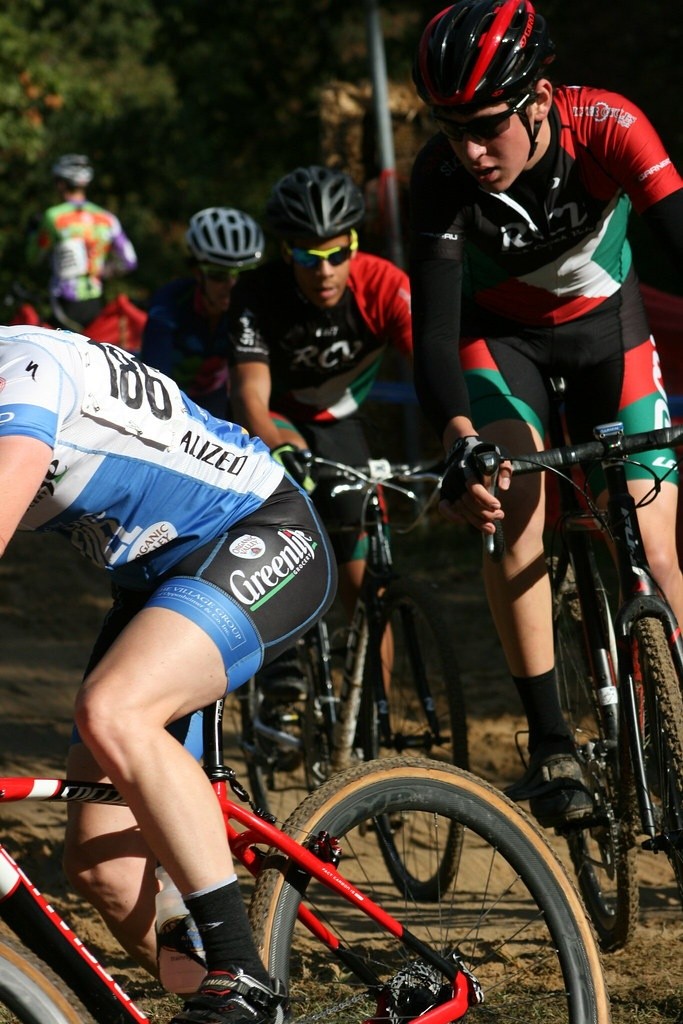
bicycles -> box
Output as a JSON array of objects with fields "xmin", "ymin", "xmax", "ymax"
[
  {"xmin": 233, "ymin": 440, "xmax": 496, "ymax": 903},
  {"xmin": 0, "ymin": 606, "xmax": 610, "ymax": 1024},
  {"xmin": 464, "ymin": 425, "xmax": 683, "ymax": 955}
]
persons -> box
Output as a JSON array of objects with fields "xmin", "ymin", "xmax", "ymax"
[
  {"xmin": 0, "ymin": 325, "xmax": 339, "ymax": 1023},
  {"xmin": 142, "ymin": 205, "xmax": 267, "ymax": 423},
  {"xmin": 222, "ymin": 164, "xmax": 410, "ymax": 745},
  {"xmin": 24, "ymin": 153, "xmax": 137, "ymax": 335},
  {"xmin": 411, "ymin": 0, "xmax": 683, "ymax": 826}
]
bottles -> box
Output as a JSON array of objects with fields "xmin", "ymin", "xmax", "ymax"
[{"xmin": 154, "ymin": 858, "xmax": 208, "ymax": 993}]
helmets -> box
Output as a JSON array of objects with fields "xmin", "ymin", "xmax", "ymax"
[
  {"xmin": 265, "ymin": 165, "xmax": 366, "ymax": 239},
  {"xmin": 411, "ymin": 0, "xmax": 558, "ymax": 115},
  {"xmin": 51, "ymin": 153, "xmax": 94, "ymax": 187},
  {"xmin": 186, "ymin": 206, "xmax": 265, "ymax": 266}
]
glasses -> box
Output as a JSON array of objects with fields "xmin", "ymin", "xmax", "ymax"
[
  {"xmin": 282, "ymin": 228, "xmax": 359, "ymax": 270},
  {"xmin": 196, "ymin": 260, "xmax": 259, "ymax": 282},
  {"xmin": 432, "ymin": 91, "xmax": 533, "ymax": 141}
]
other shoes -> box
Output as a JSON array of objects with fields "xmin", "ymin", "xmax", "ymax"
[{"xmin": 254, "ymin": 695, "xmax": 303, "ymax": 746}]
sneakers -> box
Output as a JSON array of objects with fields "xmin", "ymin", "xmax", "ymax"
[
  {"xmin": 528, "ymin": 753, "xmax": 597, "ymax": 828},
  {"xmin": 168, "ymin": 971, "xmax": 291, "ymax": 1024},
  {"xmin": 264, "ymin": 645, "xmax": 308, "ymax": 696}
]
